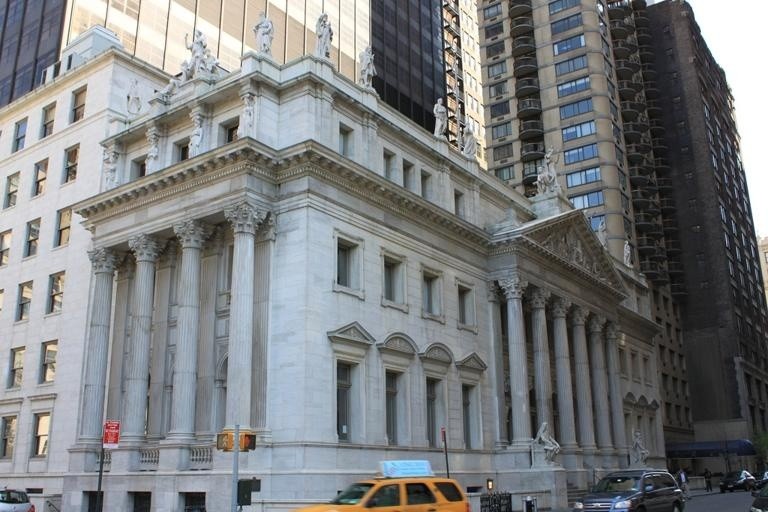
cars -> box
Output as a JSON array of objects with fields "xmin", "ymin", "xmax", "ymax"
[
  {"xmin": 718, "ymin": 470, "xmax": 768, "ymax": 512},
  {"xmin": 1, "ymin": 488, "xmax": 34, "ymax": 512},
  {"xmin": 290, "ymin": 460, "xmax": 469, "ymax": 512},
  {"xmin": 572, "ymin": 469, "xmax": 690, "ymax": 512}
]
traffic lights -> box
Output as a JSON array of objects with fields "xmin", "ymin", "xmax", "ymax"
[
  {"xmin": 215, "ymin": 429, "xmax": 256, "ymax": 453},
  {"xmin": 238, "ymin": 477, "xmax": 263, "ymax": 505}
]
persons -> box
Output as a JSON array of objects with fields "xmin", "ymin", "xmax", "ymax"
[
  {"xmin": 316, "ymin": 13, "xmax": 333, "ymax": 59},
  {"xmin": 704, "ymin": 468, "xmax": 713, "ymax": 492},
  {"xmin": 187, "ymin": 119, "xmax": 203, "ymax": 160},
  {"xmin": 599, "ymin": 221, "xmax": 608, "ymax": 250},
  {"xmin": 254, "ymin": 11, "xmax": 274, "ymax": 56},
  {"xmin": 678, "ymin": 468, "xmax": 692, "ymax": 500},
  {"xmin": 160, "ymin": 30, "xmax": 220, "ymax": 92},
  {"xmin": 463, "ymin": 121, "xmax": 478, "ymax": 160},
  {"xmin": 433, "ymin": 97, "xmax": 447, "ymax": 139},
  {"xmin": 623, "ymin": 240, "xmax": 631, "ymax": 267},
  {"xmin": 237, "ymin": 98, "xmax": 255, "ymax": 139},
  {"xmin": 358, "ymin": 45, "xmax": 377, "ymax": 90},
  {"xmin": 631, "ymin": 436, "xmax": 648, "ymax": 463},
  {"xmin": 532, "ymin": 147, "xmax": 560, "ymax": 194},
  {"xmin": 535, "ymin": 421, "xmax": 560, "ymax": 462}
]
